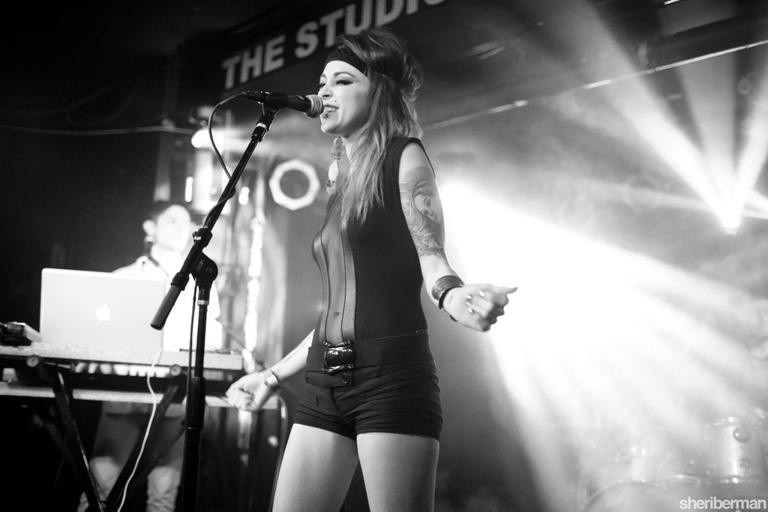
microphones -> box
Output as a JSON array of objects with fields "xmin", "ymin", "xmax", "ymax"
[{"xmin": 243, "ymin": 90, "xmax": 323, "ymax": 118}]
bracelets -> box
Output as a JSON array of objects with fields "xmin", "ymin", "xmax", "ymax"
[
  {"xmin": 263, "ymin": 368, "xmax": 282, "ymax": 393},
  {"xmin": 431, "ymin": 275, "xmax": 463, "ymax": 309}
]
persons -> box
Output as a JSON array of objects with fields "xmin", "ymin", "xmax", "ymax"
[
  {"xmin": 75, "ymin": 199, "xmax": 226, "ymax": 512},
  {"xmin": 225, "ymin": 30, "xmax": 521, "ymax": 511}
]
